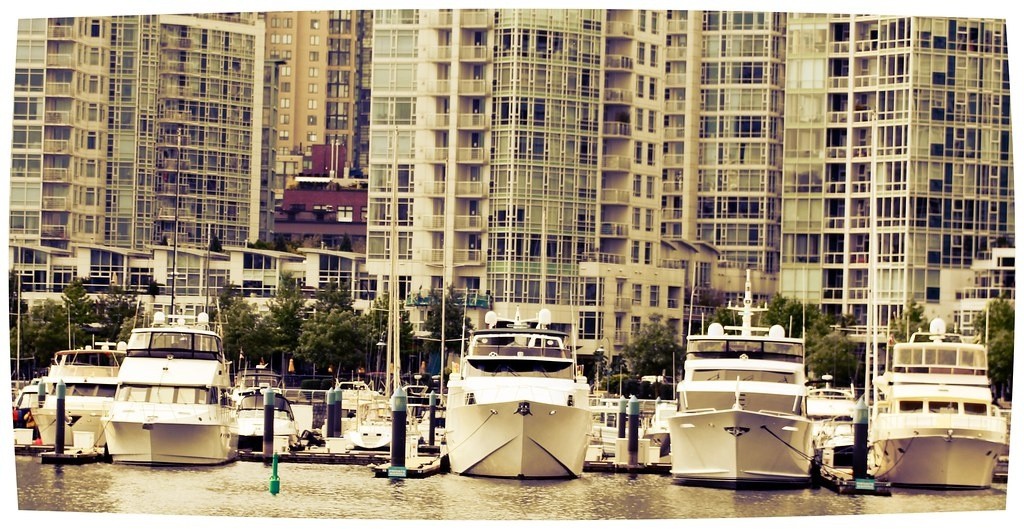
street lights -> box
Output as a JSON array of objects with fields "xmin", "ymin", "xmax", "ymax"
[
  {"xmin": 375, "ymin": 330, "xmax": 387, "ymax": 390},
  {"xmin": 594, "ymin": 337, "xmax": 611, "ymax": 427},
  {"xmin": 354, "ymin": 280, "xmax": 372, "ymax": 372}
]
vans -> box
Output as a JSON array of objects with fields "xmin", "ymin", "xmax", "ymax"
[{"xmin": 641, "ymin": 376, "xmax": 677, "ymax": 385}]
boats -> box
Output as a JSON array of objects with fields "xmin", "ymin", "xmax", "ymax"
[
  {"xmin": 344, "ymin": 400, "xmax": 412, "ymax": 449},
  {"xmin": 100, "ymin": 129, "xmax": 239, "ymax": 467},
  {"xmin": 872, "ymin": 331, "xmax": 1007, "ymax": 490},
  {"xmin": 667, "ymin": 269, "xmax": 815, "ymax": 490},
  {"xmin": 13, "ymin": 385, "xmax": 50, "ymax": 446},
  {"xmin": 30, "ymin": 350, "xmax": 126, "ymax": 447},
  {"xmin": 448, "ymin": 310, "xmax": 594, "ymax": 480},
  {"xmin": 235, "ymin": 387, "xmax": 300, "ymax": 450}
]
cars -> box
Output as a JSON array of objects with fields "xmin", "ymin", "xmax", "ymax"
[
  {"xmin": 431, "ymin": 376, "xmax": 441, "ymax": 386},
  {"xmin": 805, "ymin": 390, "xmax": 856, "ymax": 421},
  {"xmin": 355, "ymin": 372, "xmax": 387, "ymax": 391},
  {"xmin": 33, "ymin": 368, "xmax": 48, "ymax": 378},
  {"xmin": 337, "ymin": 382, "xmax": 369, "ymax": 391}
]
